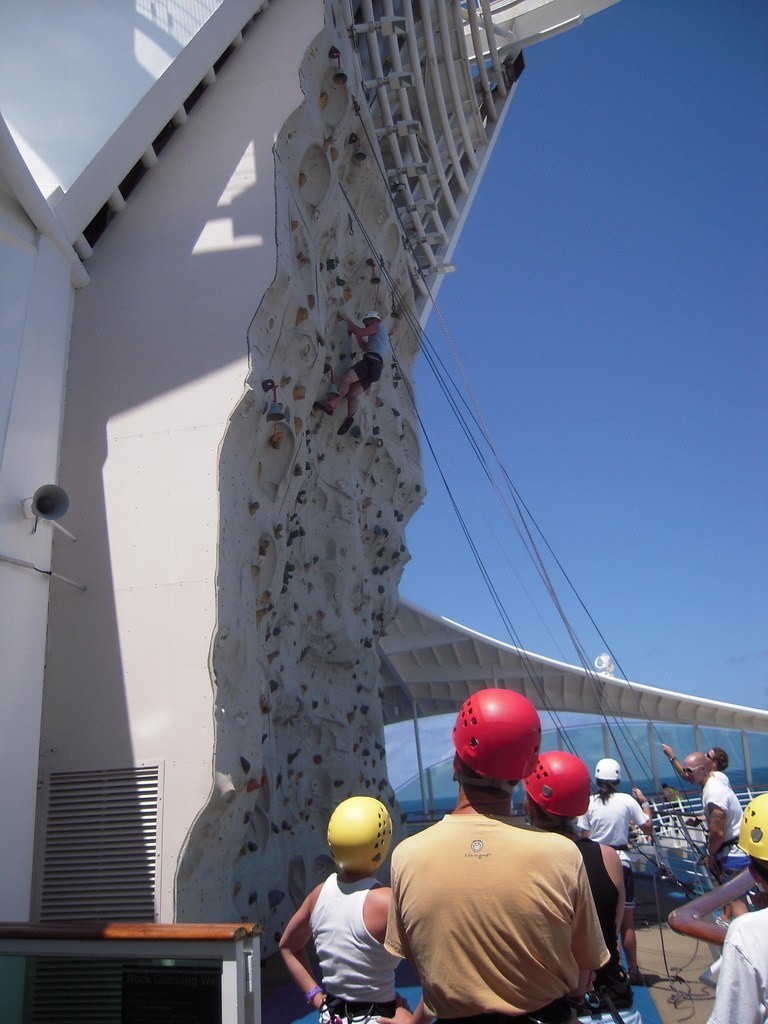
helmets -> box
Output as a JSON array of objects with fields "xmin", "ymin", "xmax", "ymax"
[
  {"xmin": 594, "ymin": 758, "xmax": 621, "ymax": 780},
  {"xmin": 363, "ymin": 311, "xmax": 380, "ymax": 322},
  {"xmin": 453, "ymin": 688, "xmax": 542, "ymax": 779},
  {"xmin": 523, "ymin": 750, "xmax": 590, "ymax": 816},
  {"xmin": 328, "ymin": 796, "xmax": 393, "ymax": 874},
  {"xmin": 737, "ymin": 793, "xmax": 768, "ymax": 860}
]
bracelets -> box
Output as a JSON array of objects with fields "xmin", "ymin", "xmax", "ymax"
[
  {"xmin": 641, "ymin": 802, "xmax": 650, "ymax": 808},
  {"xmin": 706, "ymin": 848, "xmax": 714, "ymax": 858},
  {"xmin": 304, "ymin": 985, "xmax": 323, "ymax": 1004}
]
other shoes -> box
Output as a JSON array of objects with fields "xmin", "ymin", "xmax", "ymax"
[
  {"xmin": 314, "ymin": 401, "xmax": 334, "ymax": 415},
  {"xmin": 337, "ymin": 417, "xmax": 354, "ymax": 435}
]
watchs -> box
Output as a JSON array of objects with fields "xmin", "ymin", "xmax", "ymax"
[{"xmin": 669, "ymin": 756, "xmax": 676, "ymax": 763}]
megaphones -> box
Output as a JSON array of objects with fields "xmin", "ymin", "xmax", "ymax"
[{"xmin": 32, "ymin": 484, "xmax": 70, "ymax": 521}]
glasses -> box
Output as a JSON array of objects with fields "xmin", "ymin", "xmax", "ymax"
[
  {"xmin": 707, "ymin": 752, "xmax": 713, "ymax": 761},
  {"xmin": 682, "ymin": 765, "xmax": 703, "ymax": 773}
]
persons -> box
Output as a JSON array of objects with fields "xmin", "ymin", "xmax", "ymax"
[
  {"xmin": 661, "ymin": 783, "xmax": 686, "ymax": 833},
  {"xmin": 682, "ymin": 751, "xmax": 752, "ymax": 920},
  {"xmin": 522, "ymin": 750, "xmax": 643, "ymax": 1024},
  {"xmin": 278, "ymin": 796, "xmax": 435, "ymax": 1024},
  {"xmin": 704, "ymin": 791, "xmax": 768, "ymax": 1024},
  {"xmin": 576, "ymin": 758, "xmax": 652, "ymax": 984},
  {"xmin": 661, "ymin": 743, "xmax": 732, "ymax": 928},
  {"xmin": 381, "ymin": 687, "xmax": 612, "ymax": 1024}
]
